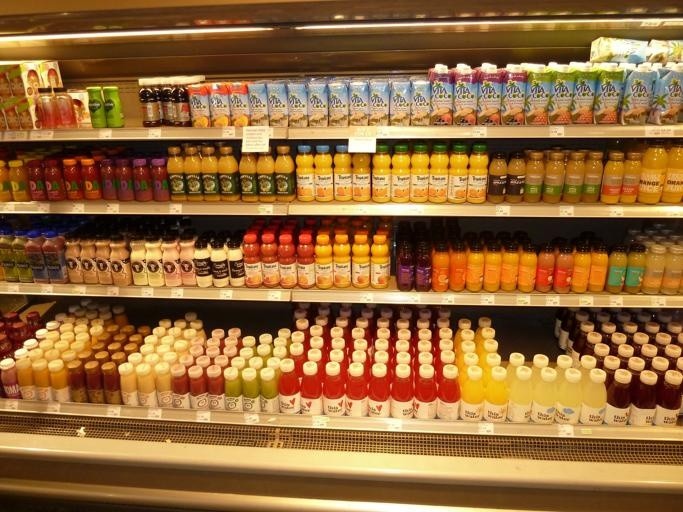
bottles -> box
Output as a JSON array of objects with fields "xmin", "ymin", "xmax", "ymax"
[
  {"xmin": 102, "ymin": 87, "xmax": 124, "ymax": 127},
  {"xmin": 2, "ymin": 141, "xmax": 683, "ymax": 208},
  {"xmin": 36, "ymin": 87, "xmax": 59, "ymax": 129},
  {"xmin": 51, "ymin": 88, "xmax": 77, "ymax": 128},
  {"xmin": 85, "ymin": 86, "xmax": 106, "ymax": 127},
  {"xmin": 136, "ymin": 75, "xmax": 206, "ymax": 128},
  {"xmin": 0, "ymin": 213, "xmax": 683, "ymax": 298},
  {"xmin": 0, "ymin": 302, "xmax": 683, "ymax": 430}
]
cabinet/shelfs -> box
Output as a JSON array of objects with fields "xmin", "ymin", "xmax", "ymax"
[{"xmin": 0, "ymin": 6, "xmax": 683, "ymax": 505}]
